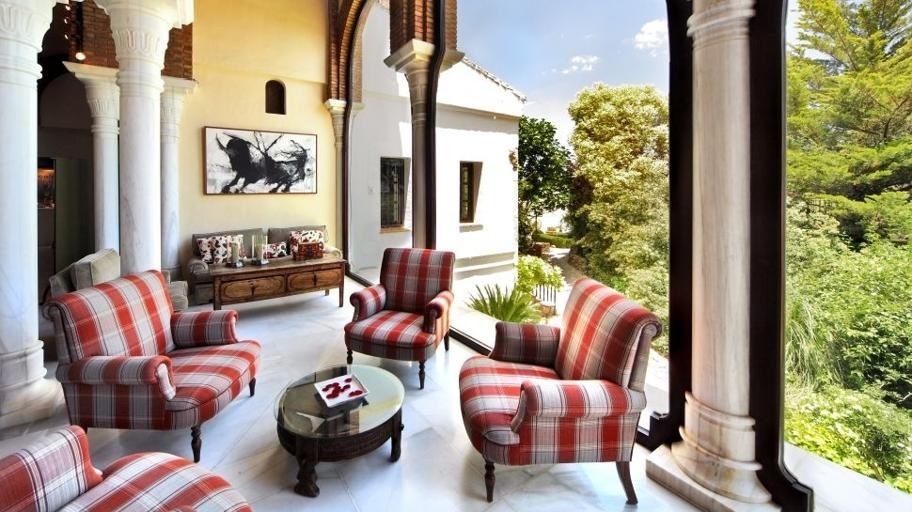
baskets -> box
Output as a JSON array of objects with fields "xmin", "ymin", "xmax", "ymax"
[{"xmin": 293, "ymin": 239, "xmax": 323, "ymax": 260}]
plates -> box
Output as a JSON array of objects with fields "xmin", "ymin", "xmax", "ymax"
[{"xmin": 314, "ymin": 373, "xmax": 369, "ymax": 409}]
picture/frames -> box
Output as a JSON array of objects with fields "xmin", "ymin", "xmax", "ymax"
[{"xmin": 202, "ymin": 126, "xmax": 318, "ymax": 196}]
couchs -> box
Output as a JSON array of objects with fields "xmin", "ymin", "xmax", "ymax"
[{"xmin": 185, "ymin": 224, "xmax": 343, "ymax": 305}]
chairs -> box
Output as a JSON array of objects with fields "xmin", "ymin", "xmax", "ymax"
[
  {"xmin": 2, "ymin": 425, "xmax": 252, "ymax": 512},
  {"xmin": 50, "ymin": 249, "xmax": 188, "ymax": 314},
  {"xmin": 344, "ymin": 246, "xmax": 456, "ymax": 389},
  {"xmin": 458, "ymin": 276, "xmax": 662, "ymax": 504},
  {"xmin": 42, "ymin": 269, "xmax": 260, "ymax": 463}
]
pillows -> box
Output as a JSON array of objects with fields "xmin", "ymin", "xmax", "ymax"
[{"xmin": 195, "ymin": 230, "xmax": 325, "ymax": 265}]
